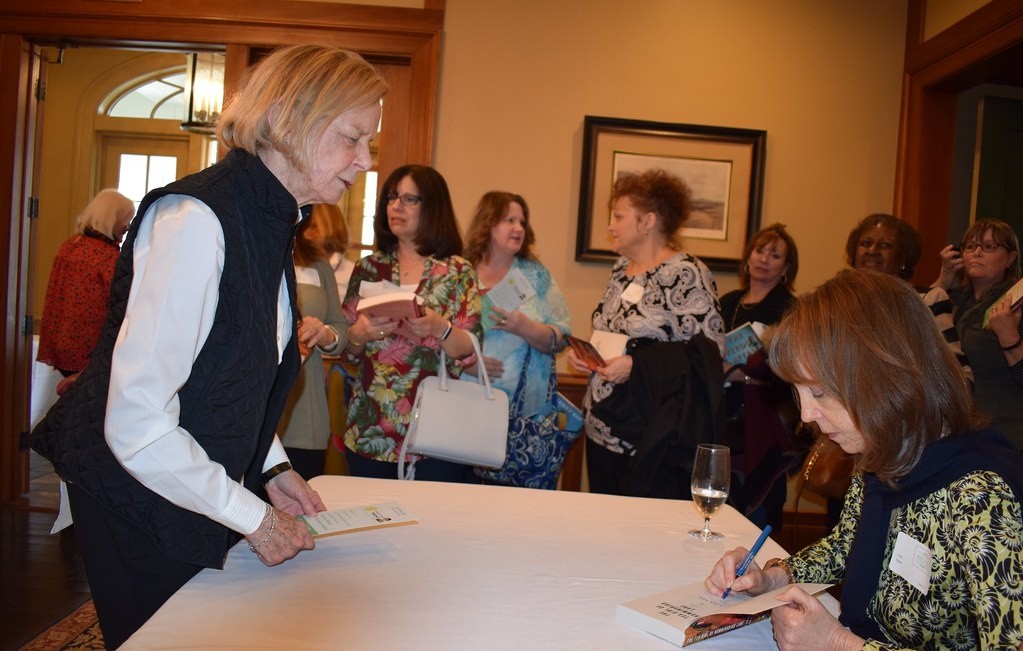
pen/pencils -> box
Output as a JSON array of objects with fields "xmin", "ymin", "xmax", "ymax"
[{"xmin": 721, "ymin": 525, "xmax": 772, "ymax": 601}]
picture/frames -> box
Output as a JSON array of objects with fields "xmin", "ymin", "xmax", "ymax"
[{"xmin": 574, "ymin": 115, "xmax": 767, "ymax": 273}]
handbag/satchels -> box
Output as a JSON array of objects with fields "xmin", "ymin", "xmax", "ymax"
[
  {"xmin": 793, "ymin": 434, "xmax": 852, "ymax": 513},
  {"xmin": 722, "ymin": 363, "xmax": 802, "ymax": 490},
  {"xmin": 475, "ymin": 327, "xmax": 584, "ymax": 489},
  {"xmin": 398, "ymin": 329, "xmax": 508, "ymax": 480}
]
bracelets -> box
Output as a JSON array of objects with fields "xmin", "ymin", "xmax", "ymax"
[
  {"xmin": 1000, "ymin": 338, "xmax": 1021, "ymax": 351},
  {"xmin": 745, "ymin": 375, "xmax": 751, "ymax": 385},
  {"xmin": 321, "ymin": 324, "xmax": 340, "ymax": 349},
  {"xmin": 249, "ymin": 504, "xmax": 275, "ymax": 552},
  {"xmin": 261, "ymin": 462, "xmax": 291, "ymax": 485}
]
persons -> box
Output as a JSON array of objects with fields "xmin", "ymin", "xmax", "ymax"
[
  {"xmin": 275, "ymin": 202, "xmax": 356, "ymax": 482},
  {"xmin": 28, "ymin": 42, "xmax": 389, "ymax": 651},
  {"xmin": 461, "ymin": 192, "xmax": 570, "ymax": 490},
  {"xmin": 716, "ymin": 213, "xmax": 1023, "ymax": 651},
  {"xmin": 341, "ymin": 165, "xmax": 483, "ymax": 482},
  {"xmin": 35, "ymin": 187, "xmax": 135, "ymax": 376},
  {"xmin": 566, "ymin": 167, "xmax": 727, "ymax": 501}
]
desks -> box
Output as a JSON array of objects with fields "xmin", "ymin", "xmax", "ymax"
[{"xmin": 115, "ymin": 474, "xmax": 852, "ymax": 651}]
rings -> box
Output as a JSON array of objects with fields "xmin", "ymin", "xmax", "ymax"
[
  {"xmin": 380, "ymin": 331, "xmax": 384, "ymax": 336},
  {"xmin": 947, "ymin": 266, "xmax": 951, "ymax": 270},
  {"xmin": 502, "ymin": 320, "xmax": 505, "ymax": 325},
  {"xmin": 991, "ymin": 308, "xmax": 997, "ymax": 311}
]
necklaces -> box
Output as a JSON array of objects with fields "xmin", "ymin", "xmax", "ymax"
[{"xmin": 399, "ymin": 254, "xmax": 423, "ymax": 275}]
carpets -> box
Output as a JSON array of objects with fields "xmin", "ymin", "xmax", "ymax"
[{"xmin": 12, "ymin": 595, "xmax": 107, "ymax": 651}]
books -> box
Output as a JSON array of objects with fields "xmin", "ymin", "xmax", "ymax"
[
  {"xmin": 615, "ymin": 582, "xmax": 836, "ymax": 648},
  {"xmin": 356, "ymin": 293, "xmax": 427, "ymax": 344},
  {"xmin": 983, "ymin": 279, "xmax": 1023, "ymax": 330},
  {"xmin": 560, "ymin": 329, "xmax": 631, "ymax": 373},
  {"xmin": 723, "ymin": 322, "xmax": 763, "ymax": 365},
  {"xmin": 532, "ymin": 391, "xmax": 584, "ymax": 431}
]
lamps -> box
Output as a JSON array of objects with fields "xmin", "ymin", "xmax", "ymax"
[{"xmin": 180, "ymin": 52, "xmax": 225, "ymax": 135}]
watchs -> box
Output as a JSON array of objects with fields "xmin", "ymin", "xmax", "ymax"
[{"xmin": 435, "ymin": 320, "xmax": 453, "ymax": 341}]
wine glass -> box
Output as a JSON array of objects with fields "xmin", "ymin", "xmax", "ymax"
[{"xmin": 688, "ymin": 444, "xmax": 731, "ymax": 542}]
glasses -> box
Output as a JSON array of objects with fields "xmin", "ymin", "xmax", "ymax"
[
  {"xmin": 387, "ymin": 193, "xmax": 420, "ymax": 205},
  {"xmin": 963, "ymin": 241, "xmax": 1008, "ymax": 252}
]
